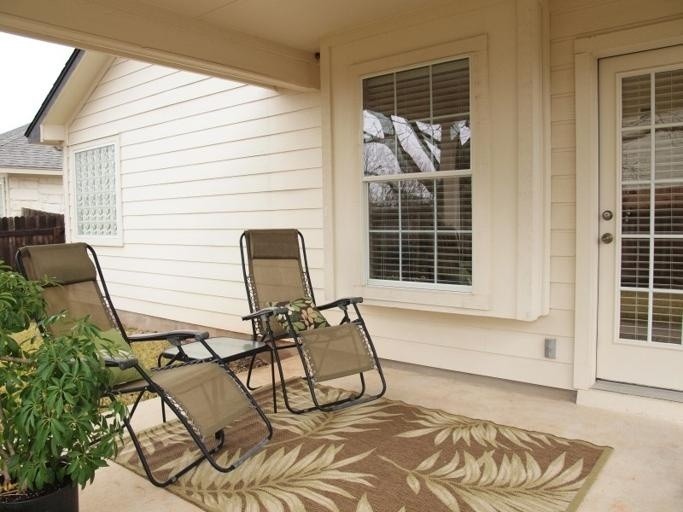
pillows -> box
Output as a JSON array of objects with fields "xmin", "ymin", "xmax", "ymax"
[
  {"xmin": 268, "ymin": 297, "xmax": 331, "ymax": 333},
  {"xmin": 67, "ymin": 328, "xmax": 154, "ymax": 389}
]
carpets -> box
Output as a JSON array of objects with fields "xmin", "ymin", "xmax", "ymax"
[{"xmin": 104, "ymin": 377, "xmax": 614, "ymax": 512}]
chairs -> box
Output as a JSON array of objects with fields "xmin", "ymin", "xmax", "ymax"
[
  {"xmin": 16, "ymin": 242, "xmax": 273, "ymax": 487},
  {"xmin": 239, "ymin": 229, "xmax": 386, "ymax": 414}
]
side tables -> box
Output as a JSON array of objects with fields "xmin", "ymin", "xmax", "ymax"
[{"xmin": 158, "ymin": 336, "xmax": 277, "ymax": 423}]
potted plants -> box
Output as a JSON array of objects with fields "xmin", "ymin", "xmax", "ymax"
[{"xmin": 0, "ymin": 260, "xmax": 128, "ymax": 512}]
